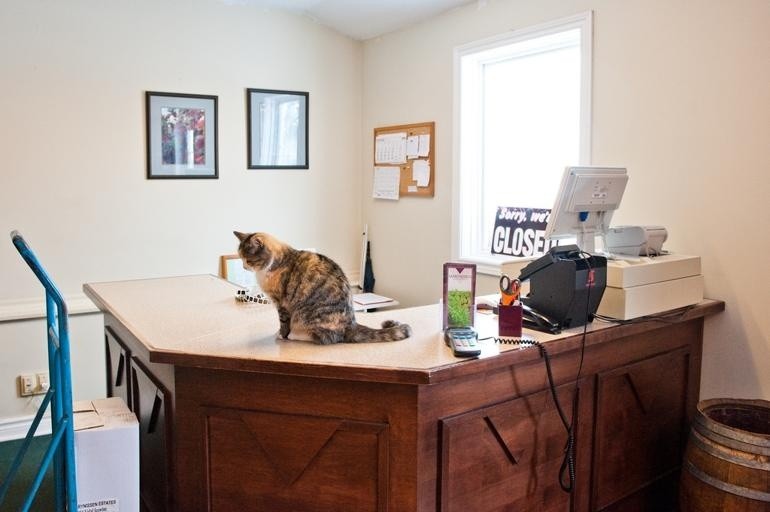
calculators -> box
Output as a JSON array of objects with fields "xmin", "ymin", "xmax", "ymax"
[{"xmin": 445, "ymin": 325, "xmax": 481, "ymax": 357}]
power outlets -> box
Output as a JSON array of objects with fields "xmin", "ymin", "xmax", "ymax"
[{"xmin": 21, "ymin": 373, "xmax": 50, "ymax": 397}]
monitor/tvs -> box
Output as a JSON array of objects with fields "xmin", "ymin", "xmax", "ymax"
[{"xmin": 543, "ymin": 166, "xmax": 629, "ymax": 258}]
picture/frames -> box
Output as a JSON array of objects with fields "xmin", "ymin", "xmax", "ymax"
[
  {"xmin": 244, "ymin": 86, "xmax": 312, "ymax": 171},
  {"xmin": 144, "ymin": 90, "xmax": 218, "ymax": 180},
  {"xmin": 222, "ymin": 255, "xmax": 258, "ymax": 289},
  {"xmin": 442, "ymin": 263, "xmax": 477, "ymax": 332}
]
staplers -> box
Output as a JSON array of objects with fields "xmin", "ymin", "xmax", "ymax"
[{"xmin": 521, "ymin": 304, "xmax": 562, "ymax": 335}]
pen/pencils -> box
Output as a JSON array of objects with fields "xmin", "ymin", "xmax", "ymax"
[{"xmin": 510, "ymin": 279, "xmax": 516, "ymax": 305}]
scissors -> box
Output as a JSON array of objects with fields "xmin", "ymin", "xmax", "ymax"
[{"xmin": 499, "ymin": 274, "xmax": 521, "ymax": 305}]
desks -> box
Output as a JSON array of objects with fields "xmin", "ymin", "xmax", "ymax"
[
  {"xmin": 349, "ymin": 292, "xmax": 400, "ymax": 314},
  {"xmin": 79, "ymin": 271, "xmax": 726, "ymax": 512}
]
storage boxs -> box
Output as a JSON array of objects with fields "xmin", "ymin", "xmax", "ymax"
[{"xmin": 72, "ymin": 396, "xmax": 145, "ymax": 512}]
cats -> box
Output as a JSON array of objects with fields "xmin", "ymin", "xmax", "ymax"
[{"xmin": 232, "ymin": 230, "xmax": 412, "ymax": 346}]
